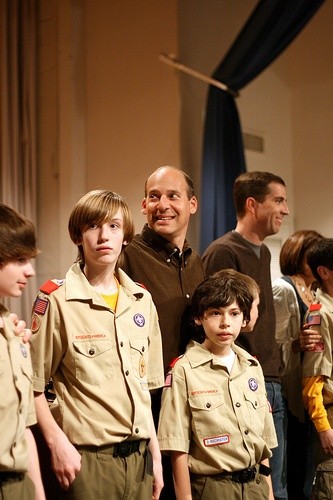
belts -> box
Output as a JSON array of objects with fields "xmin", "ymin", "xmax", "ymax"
[
  {"xmin": 210, "ymin": 464, "xmax": 272, "ymax": 483},
  {"xmin": 95, "ymin": 438, "xmax": 141, "ymax": 458}
]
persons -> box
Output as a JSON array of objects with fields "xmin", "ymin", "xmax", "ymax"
[
  {"xmin": 272, "ymin": 230, "xmax": 324, "ymax": 500},
  {"xmin": 0, "ymin": 204, "xmax": 45, "ymax": 499},
  {"xmin": 199, "ymin": 172, "xmax": 290, "ymax": 500},
  {"xmin": 30, "ymin": 189, "xmax": 164, "ymax": 499},
  {"xmin": 303, "ymin": 238, "xmax": 333, "ymax": 500},
  {"xmin": 217, "ymin": 269, "xmax": 262, "ymax": 332},
  {"xmin": 117, "ymin": 164, "xmax": 206, "ymax": 371},
  {"xmin": 157, "ymin": 273, "xmax": 278, "ymax": 500}
]
